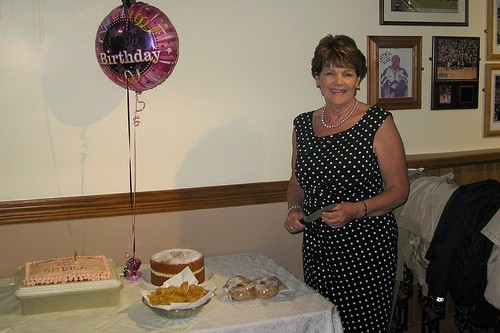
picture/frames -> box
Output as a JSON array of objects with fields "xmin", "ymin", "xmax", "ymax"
[
  {"xmin": 379, "ymin": 0, "xmax": 469, "ymax": 27},
  {"xmin": 431, "ymin": 36, "xmax": 480, "ymax": 110},
  {"xmin": 483, "ymin": 63, "xmax": 500, "ymax": 138},
  {"xmin": 366, "ymin": 36, "xmax": 423, "ymax": 110},
  {"xmin": 484, "ymin": 0, "xmax": 500, "ymax": 61}
]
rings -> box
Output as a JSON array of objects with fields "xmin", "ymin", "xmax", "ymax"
[
  {"xmin": 291, "ymin": 227, "xmax": 293, "ymax": 229},
  {"xmin": 332, "ymin": 225, "xmax": 335, "ymax": 228}
]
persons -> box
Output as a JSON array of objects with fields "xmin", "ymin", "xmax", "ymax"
[
  {"xmin": 283, "ymin": 34, "xmax": 410, "ymax": 333},
  {"xmin": 380, "ymin": 39, "xmax": 478, "ymax": 104}
]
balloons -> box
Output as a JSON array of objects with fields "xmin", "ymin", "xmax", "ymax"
[{"xmin": 94, "ymin": 1, "xmax": 180, "ymax": 95}]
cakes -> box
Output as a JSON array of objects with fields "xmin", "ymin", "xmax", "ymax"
[
  {"xmin": 22, "ymin": 255, "xmax": 112, "ymax": 286},
  {"xmin": 150, "ymin": 248, "xmax": 205, "ymax": 286}
]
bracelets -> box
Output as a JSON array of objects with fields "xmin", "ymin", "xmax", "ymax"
[
  {"xmin": 362, "ymin": 200, "xmax": 368, "ymax": 218},
  {"xmin": 285, "ymin": 206, "xmax": 302, "ymax": 215}
]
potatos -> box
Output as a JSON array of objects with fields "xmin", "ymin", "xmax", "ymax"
[{"xmin": 149, "ymin": 281, "xmax": 207, "ymax": 305}]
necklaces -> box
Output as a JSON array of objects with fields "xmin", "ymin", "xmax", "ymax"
[{"xmin": 321, "ymin": 100, "xmax": 359, "ymax": 129}]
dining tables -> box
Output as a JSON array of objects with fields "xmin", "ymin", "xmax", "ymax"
[{"xmin": 0, "ymin": 252, "xmax": 343, "ymax": 333}]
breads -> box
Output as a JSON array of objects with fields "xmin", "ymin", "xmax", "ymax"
[{"xmin": 223, "ymin": 275, "xmax": 281, "ymax": 301}]
